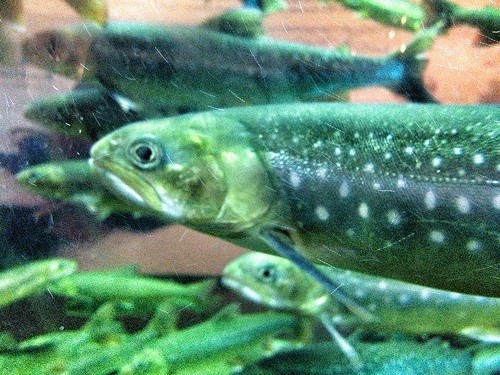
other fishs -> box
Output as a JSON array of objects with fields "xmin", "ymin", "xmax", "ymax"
[{"xmin": 0, "ymin": 0, "xmax": 500, "ymax": 375}]
